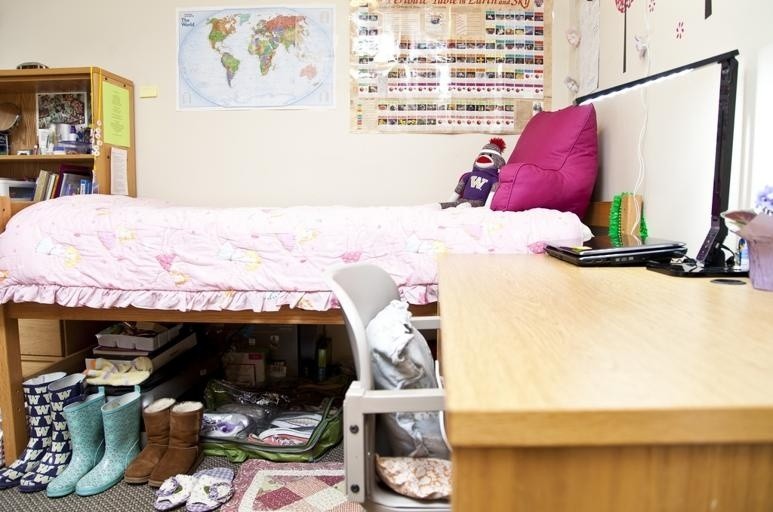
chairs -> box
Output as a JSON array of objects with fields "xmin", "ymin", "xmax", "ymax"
[{"xmin": 322, "ymin": 262, "xmax": 452, "ymax": 512}]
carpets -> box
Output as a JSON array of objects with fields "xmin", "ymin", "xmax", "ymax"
[{"xmin": 220, "ymin": 459, "xmax": 368, "ymax": 512}]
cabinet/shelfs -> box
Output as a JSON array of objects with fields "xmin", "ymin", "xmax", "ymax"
[{"xmin": 0, "ymin": 67, "xmax": 137, "ymax": 218}]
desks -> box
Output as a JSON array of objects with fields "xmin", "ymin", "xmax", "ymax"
[{"xmin": 437, "ymin": 250, "xmax": 773, "ymax": 510}]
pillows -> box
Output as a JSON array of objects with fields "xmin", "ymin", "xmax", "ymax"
[{"xmin": 490, "ymin": 104, "xmax": 598, "ymax": 218}]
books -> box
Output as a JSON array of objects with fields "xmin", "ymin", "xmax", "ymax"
[{"xmin": 28, "ymin": 164, "xmax": 94, "ymax": 202}]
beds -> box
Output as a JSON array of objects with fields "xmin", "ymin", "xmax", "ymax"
[{"xmin": 0, "ymin": 191, "xmax": 645, "ymax": 471}]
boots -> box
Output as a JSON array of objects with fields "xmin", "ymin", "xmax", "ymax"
[{"xmin": 0, "ymin": 372, "xmax": 203, "ymax": 498}]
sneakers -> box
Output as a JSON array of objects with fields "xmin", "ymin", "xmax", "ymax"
[{"xmin": 200, "ymin": 403, "xmax": 322, "ymax": 448}]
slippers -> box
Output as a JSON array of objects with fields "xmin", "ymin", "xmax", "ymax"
[{"xmin": 153, "ymin": 467, "xmax": 234, "ymax": 512}]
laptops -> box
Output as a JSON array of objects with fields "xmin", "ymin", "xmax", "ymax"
[{"xmin": 542, "ymin": 237, "xmax": 688, "ymax": 267}]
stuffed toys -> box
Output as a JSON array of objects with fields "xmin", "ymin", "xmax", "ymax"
[{"xmin": 438, "ymin": 136, "xmax": 507, "ymax": 210}]
